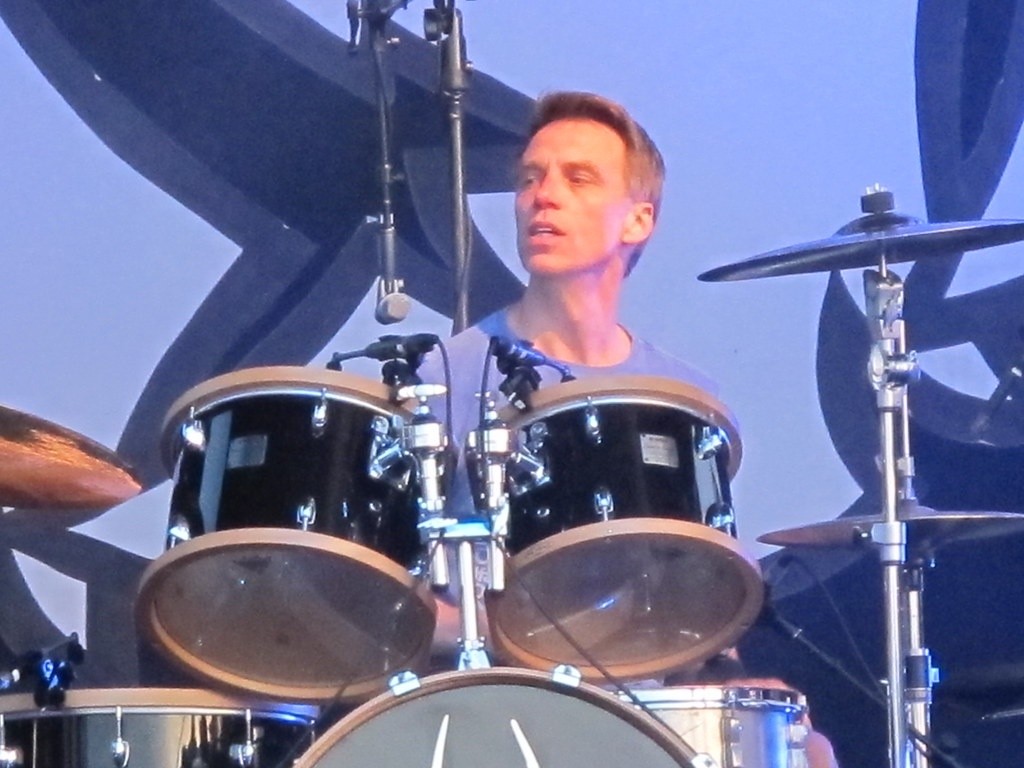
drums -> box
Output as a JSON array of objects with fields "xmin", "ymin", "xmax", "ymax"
[
  {"xmin": 130, "ymin": 363, "xmax": 442, "ymax": 705},
  {"xmin": 605, "ymin": 676, "xmax": 812, "ymax": 768},
  {"xmin": 0, "ymin": 683, "xmax": 325, "ymax": 768},
  {"xmin": 291, "ymin": 664, "xmax": 723, "ymax": 768},
  {"xmin": 469, "ymin": 373, "xmax": 766, "ymax": 683}
]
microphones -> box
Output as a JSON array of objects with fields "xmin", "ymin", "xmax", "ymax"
[
  {"xmin": 375, "ymin": 293, "xmax": 412, "ymax": 325},
  {"xmin": 762, "ymin": 557, "xmax": 792, "ymax": 597}
]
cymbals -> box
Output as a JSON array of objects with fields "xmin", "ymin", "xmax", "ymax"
[
  {"xmin": 0, "ymin": 402, "xmax": 145, "ymax": 513},
  {"xmin": 695, "ymin": 219, "xmax": 1024, "ymax": 283},
  {"xmin": 755, "ymin": 504, "xmax": 1024, "ymax": 554}
]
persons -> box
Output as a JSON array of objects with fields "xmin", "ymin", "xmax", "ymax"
[{"xmin": 416, "ymin": 92, "xmax": 841, "ymax": 768}]
